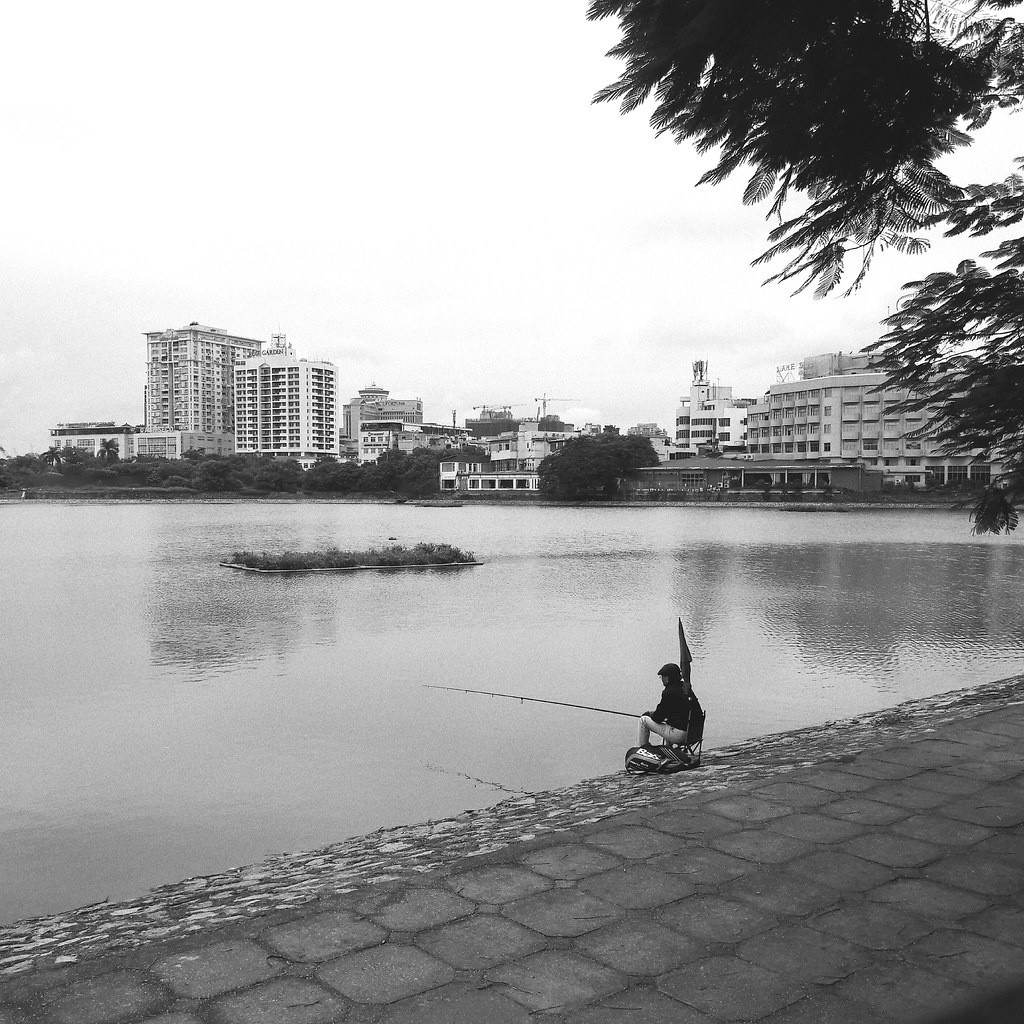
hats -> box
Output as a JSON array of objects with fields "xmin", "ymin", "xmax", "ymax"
[{"xmin": 658, "ymin": 663, "xmax": 680, "ymax": 675}]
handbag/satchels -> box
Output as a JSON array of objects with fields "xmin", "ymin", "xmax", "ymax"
[{"xmin": 625, "ymin": 742, "xmax": 700, "ymax": 774}]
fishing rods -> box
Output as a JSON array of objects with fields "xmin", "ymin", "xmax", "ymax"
[{"xmin": 423, "ymin": 684, "xmax": 644, "ymax": 719}]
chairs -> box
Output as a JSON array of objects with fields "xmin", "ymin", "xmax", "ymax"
[{"xmin": 661, "ymin": 710, "xmax": 707, "ymax": 765}]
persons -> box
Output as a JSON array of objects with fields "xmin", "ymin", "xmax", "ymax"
[{"xmin": 637, "ymin": 663, "xmax": 703, "ymax": 748}]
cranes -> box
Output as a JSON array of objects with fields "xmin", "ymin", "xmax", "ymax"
[{"xmin": 535, "ymin": 393, "xmax": 582, "ymax": 420}]
